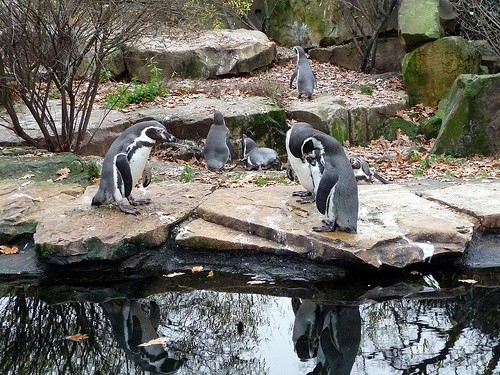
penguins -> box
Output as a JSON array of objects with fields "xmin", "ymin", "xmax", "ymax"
[
  {"xmin": 98, "ymin": 299, "xmax": 190, "ymax": 375},
  {"xmin": 349, "ymin": 156, "xmax": 392, "ymax": 186},
  {"xmin": 284, "ymin": 117, "xmax": 325, "ymax": 203},
  {"xmin": 306, "ymin": 306, "xmax": 362, "ymax": 375},
  {"xmin": 90, "ymin": 120, "xmax": 177, "ymax": 217},
  {"xmin": 290, "ymin": 45, "xmax": 318, "ymax": 100},
  {"xmin": 239, "ymin": 134, "xmax": 283, "ymax": 171},
  {"xmin": 202, "ymin": 111, "xmax": 238, "ymax": 171},
  {"xmin": 301, "ymin": 134, "xmax": 358, "ymax": 233},
  {"xmin": 291, "ymin": 297, "xmax": 331, "ymax": 362}
]
